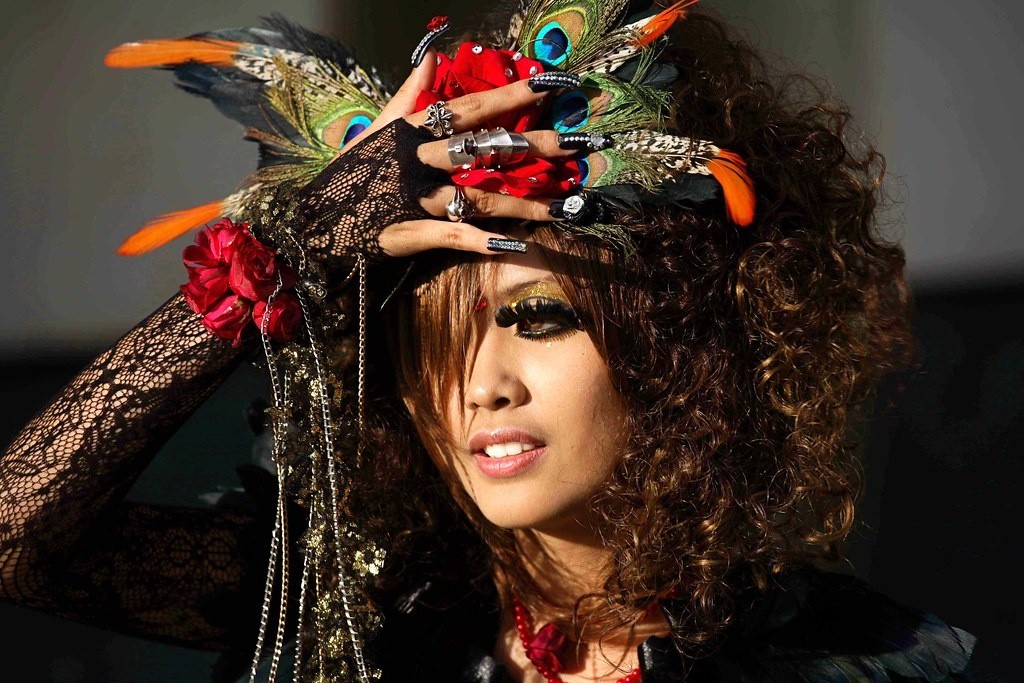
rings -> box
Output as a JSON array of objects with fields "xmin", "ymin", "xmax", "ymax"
[
  {"xmin": 424, "ymin": 101, "xmax": 454, "ymax": 138},
  {"xmin": 448, "ymin": 126, "xmax": 529, "ymax": 170},
  {"xmin": 444, "ymin": 185, "xmax": 477, "ymax": 222}
]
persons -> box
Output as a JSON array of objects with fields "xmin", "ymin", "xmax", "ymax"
[{"xmin": 0, "ymin": 0, "xmax": 1024, "ymax": 683}]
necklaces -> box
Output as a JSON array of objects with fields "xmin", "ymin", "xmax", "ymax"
[{"xmin": 513, "ymin": 596, "xmax": 642, "ymax": 683}]
